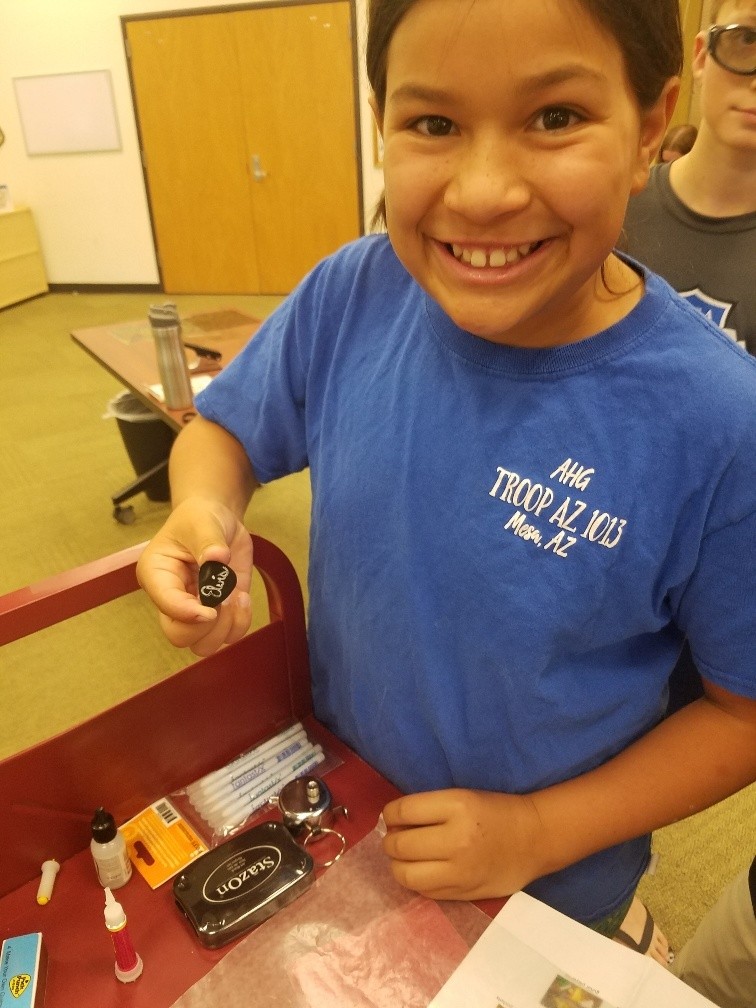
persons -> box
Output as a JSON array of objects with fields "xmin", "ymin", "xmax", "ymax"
[
  {"xmin": 614, "ymin": 0, "xmax": 756, "ymax": 359},
  {"xmin": 135, "ymin": 0, "xmax": 756, "ymax": 965},
  {"xmin": 678, "ymin": 857, "xmax": 756, "ymax": 1008}
]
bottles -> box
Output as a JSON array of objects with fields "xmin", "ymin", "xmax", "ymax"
[{"xmin": 91, "ymin": 808, "xmax": 131, "ymax": 889}]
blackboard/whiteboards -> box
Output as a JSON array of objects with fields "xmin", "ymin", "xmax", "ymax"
[{"xmin": 12, "ymin": 70, "xmax": 124, "ymax": 156}]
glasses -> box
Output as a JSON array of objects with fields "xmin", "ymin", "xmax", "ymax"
[{"xmin": 706, "ymin": 25, "xmax": 756, "ymax": 75}]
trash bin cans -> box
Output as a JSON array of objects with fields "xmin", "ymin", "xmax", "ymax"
[{"xmin": 110, "ymin": 390, "xmax": 176, "ymax": 503}]
